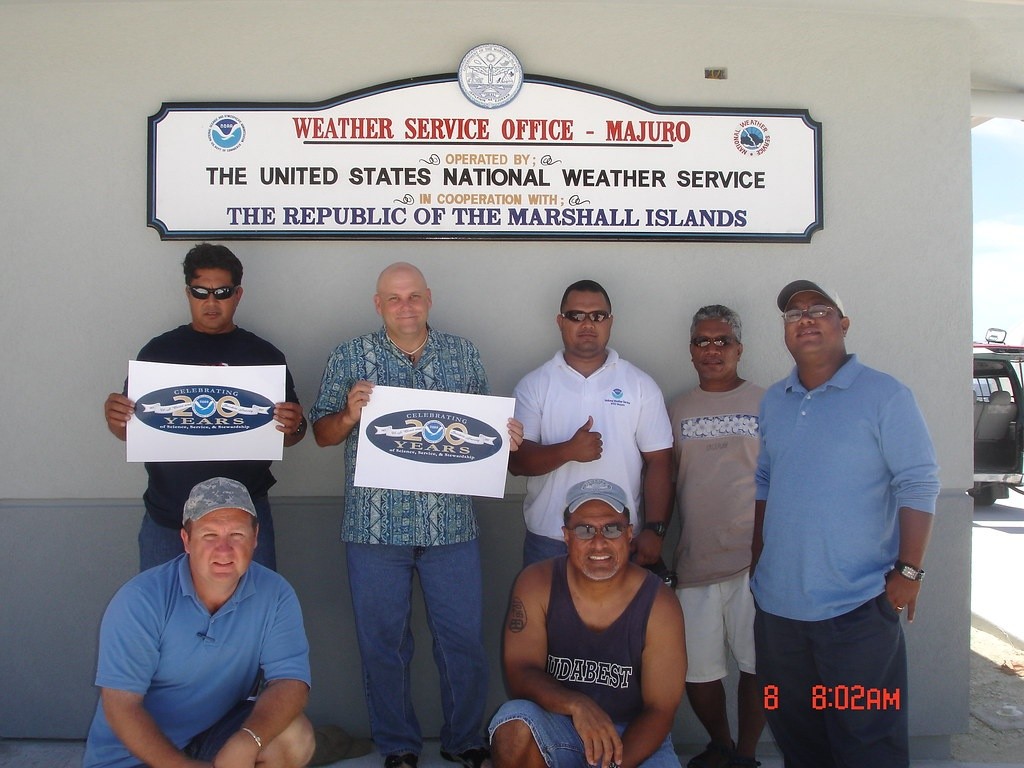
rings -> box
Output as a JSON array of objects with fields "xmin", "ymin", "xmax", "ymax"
[{"xmin": 898, "ymin": 606, "xmax": 906, "ymax": 611}]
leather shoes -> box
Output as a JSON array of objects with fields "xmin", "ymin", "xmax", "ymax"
[
  {"xmin": 385, "ymin": 753, "xmax": 418, "ymax": 768},
  {"xmin": 440, "ymin": 747, "xmax": 491, "ymax": 768}
]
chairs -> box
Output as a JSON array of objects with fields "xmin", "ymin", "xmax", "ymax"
[
  {"xmin": 973, "ymin": 390, "xmax": 984, "ymax": 431},
  {"xmin": 975, "ymin": 392, "xmax": 1016, "ymax": 442}
]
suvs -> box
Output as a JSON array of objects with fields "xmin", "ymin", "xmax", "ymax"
[{"xmin": 968, "ymin": 327, "xmax": 1024, "ymax": 505}]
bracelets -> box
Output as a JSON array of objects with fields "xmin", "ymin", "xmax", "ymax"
[
  {"xmin": 241, "ymin": 727, "xmax": 262, "ymax": 748},
  {"xmin": 291, "ymin": 416, "xmax": 306, "ymax": 436}
]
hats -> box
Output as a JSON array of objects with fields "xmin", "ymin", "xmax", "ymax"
[
  {"xmin": 566, "ymin": 478, "xmax": 630, "ymax": 513},
  {"xmin": 183, "ymin": 477, "xmax": 257, "ymax": 525}
]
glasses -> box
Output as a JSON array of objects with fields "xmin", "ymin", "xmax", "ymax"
[
  {"xmin": 568, "ymin": 523, "xmax": 628, "ymax": 539},
  {"xmin": 562, "ymin": 311, "xmax": 612, "ymax": 322},
  {"xmin": 692, "ymin": 337, "xmax": 740, "ymax": 347},
  {"xmin": 188, "ymin": 285, "xmax": 239, "ymax": 300},
  {"xmin": 781, "ymin": 305, "xmax": 833, "ymax": 323}
]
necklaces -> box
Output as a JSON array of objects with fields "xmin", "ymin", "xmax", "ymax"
[{"xmin": 384, "ymin": 328, "xmax": 428, "ymax": 362}]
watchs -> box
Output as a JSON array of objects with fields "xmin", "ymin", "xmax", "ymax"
[
  {"xmin": 643, "ymin": 520, "xmax": 667, "ymax": 537},
  {"xmin": 893, "ymin": 559, "xmax": 926, "ymax": 582}
]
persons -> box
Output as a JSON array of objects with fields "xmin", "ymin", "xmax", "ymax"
[
  {"xmin": 671, "ymin": 304, "xmax": 772, "ymax": 768},
  {"xmin": 313, "ymin": 262, "xmax": 526, "ymax": 768},
  {"xmin": 81, "ymin": 476, "xmax": 316, "ymax": 768},
  {"xmin": 486, "ymin": 478, "xmax": 688, "ymax": 768},
  {"xmin": 507, "ymin": 279, "xmax": 675, "ymax": 573},
  {"xmin": 106, "ymin": 242, "xmax": 307, "ymax": 576},
  {"xmin": 750, "ymin": 280, "xmax": 940, "ymax": 768}
]
